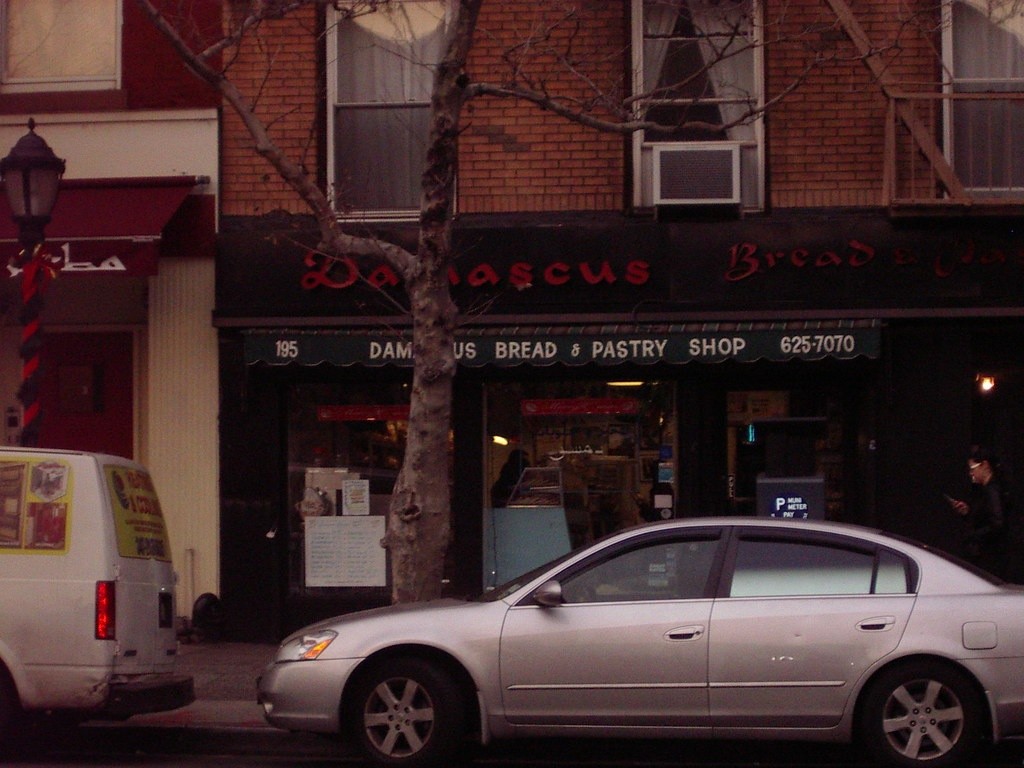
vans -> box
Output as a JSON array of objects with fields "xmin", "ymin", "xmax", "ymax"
[{"xmin": 0, "ymin": 445, "xmax": 198, "ymax": 768}]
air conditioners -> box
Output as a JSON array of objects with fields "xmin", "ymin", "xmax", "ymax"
[{"xmin": 651, "ymin": 143, "xmax": 742, "ymax": 206}]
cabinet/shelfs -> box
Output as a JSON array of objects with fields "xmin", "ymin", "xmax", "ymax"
[{"xmin": 506, "ymin": 466, "xmax": 564, "ymax": 508}]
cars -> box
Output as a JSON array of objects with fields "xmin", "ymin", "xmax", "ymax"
[{"xmin": 255, "ymin": 516, "xmax": 1024, "ymax": 768}]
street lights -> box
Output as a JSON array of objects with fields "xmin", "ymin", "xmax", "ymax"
[{"xmin": 0, "ymin": 115, "xmax": 68, "ymax": 447}]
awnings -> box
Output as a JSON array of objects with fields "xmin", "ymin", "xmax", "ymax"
[
  {"xmin": 0, "ymin": 167, "xmax": 211, "ymax": 280},
  {"xmin": 243, "ymin": 317, "xmax": 883, "ymax": 368}
]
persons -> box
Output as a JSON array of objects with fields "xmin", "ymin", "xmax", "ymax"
[
  {"xmin": 736, "ymin": 442, "xmax": 770, "ymax": 516},
  {"xmin": 951, "ymin": 447, "xmax": 1012, "ymax": 578},
  {"xmin": 491, "ymin": 449, "xmax": 532, "ymax": 508}
]
